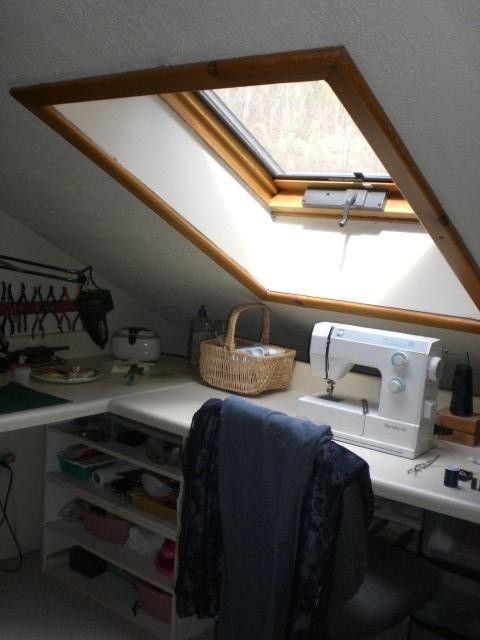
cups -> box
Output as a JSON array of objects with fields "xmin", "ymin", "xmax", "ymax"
[{"xmin": 15, "ymin": 368, "xmax": 31, "ymax": 387}]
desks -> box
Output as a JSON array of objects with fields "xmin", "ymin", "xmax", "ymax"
[{"xmin": 118, "ymin": 359, "xmax": 480, "ymax": 640}]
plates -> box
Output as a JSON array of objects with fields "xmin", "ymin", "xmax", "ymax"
[{"xmin": 31, "ymin": 364, "xmax": 104, "ymax": 384}]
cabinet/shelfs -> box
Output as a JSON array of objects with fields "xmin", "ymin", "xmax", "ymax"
[{"xmin": 42, "ymin": 422, "xmax": 214, "ymax": 640}]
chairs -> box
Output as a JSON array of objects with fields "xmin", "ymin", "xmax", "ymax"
[{"xmin": 182, "ymin": 397, "xmax": 441, "ymax": 640}]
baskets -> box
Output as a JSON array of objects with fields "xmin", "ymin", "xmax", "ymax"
[{"xmin": 198, "ymin": 302, "xmax": 296, "ymax": 396}]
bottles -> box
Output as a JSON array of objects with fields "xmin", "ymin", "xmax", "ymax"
[{"xmin": 184, "ymin": 302, "xmax": 215, "ymax": 368}]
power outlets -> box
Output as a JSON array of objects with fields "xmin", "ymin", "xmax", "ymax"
[{"xmin": 0, "ymin": 449, "xmax": 15, "ymax": 473}]
